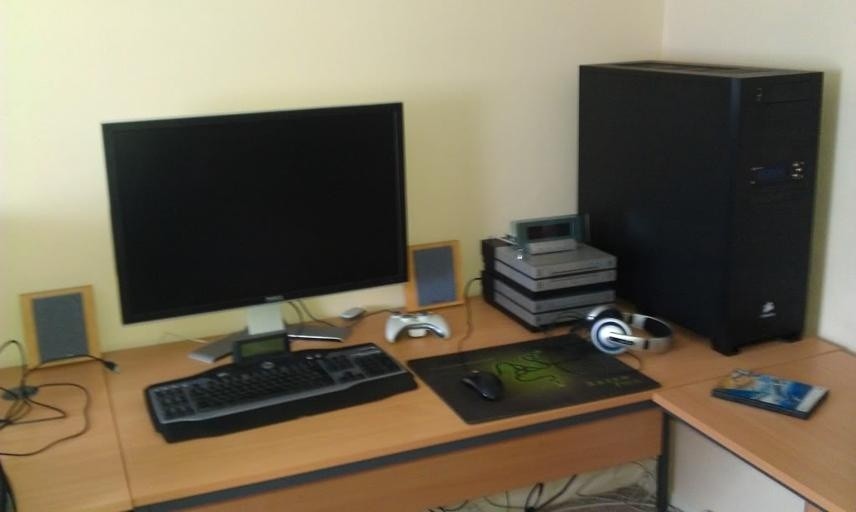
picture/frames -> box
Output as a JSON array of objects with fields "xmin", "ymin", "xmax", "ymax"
[
  {"xmin": 404, "ymin": 236, "xmax": 466, "ymax": 314},
  {"xmin": 17, "ymin": 282, "xmax": 104, "ymax": 370}
]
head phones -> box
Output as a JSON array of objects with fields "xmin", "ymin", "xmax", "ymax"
[{"xmin": 586, "ymin": 305, "xmax": 674, "ymax": 356}]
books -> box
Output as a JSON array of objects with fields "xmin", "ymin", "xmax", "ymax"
[{"xmin": 712, "ymin": 368, "xmax": 829, "ymax": 420}]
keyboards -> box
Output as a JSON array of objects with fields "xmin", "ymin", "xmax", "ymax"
[{"xmin": 143, "ymin": 341, "xmax": 419, "ymax": 444}]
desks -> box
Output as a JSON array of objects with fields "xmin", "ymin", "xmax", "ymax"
[
  {"xmin": 103, "ymin": 296, "xmax": 847, "ymax": 512},
  {"xmin": 2, "ymin": 359, "xmax": 134, "ymax": 512},
  {"xmin": 654, "ymin": 350, "xmax": 856, "ymax": 512}
]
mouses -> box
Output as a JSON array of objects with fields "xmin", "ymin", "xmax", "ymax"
[{"xmin": 461, "ymin": 367, "xmax": 504, "ymax": 402}]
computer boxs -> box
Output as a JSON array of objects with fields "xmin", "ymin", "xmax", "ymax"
[{"xmin": 577, "ymin": 59, "xmax": 825, "ymax": 354}]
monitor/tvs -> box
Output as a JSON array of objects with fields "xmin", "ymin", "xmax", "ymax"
[{"xmin": 101, "ymin": 101, "xmax": 410, "ymax": 363}]
